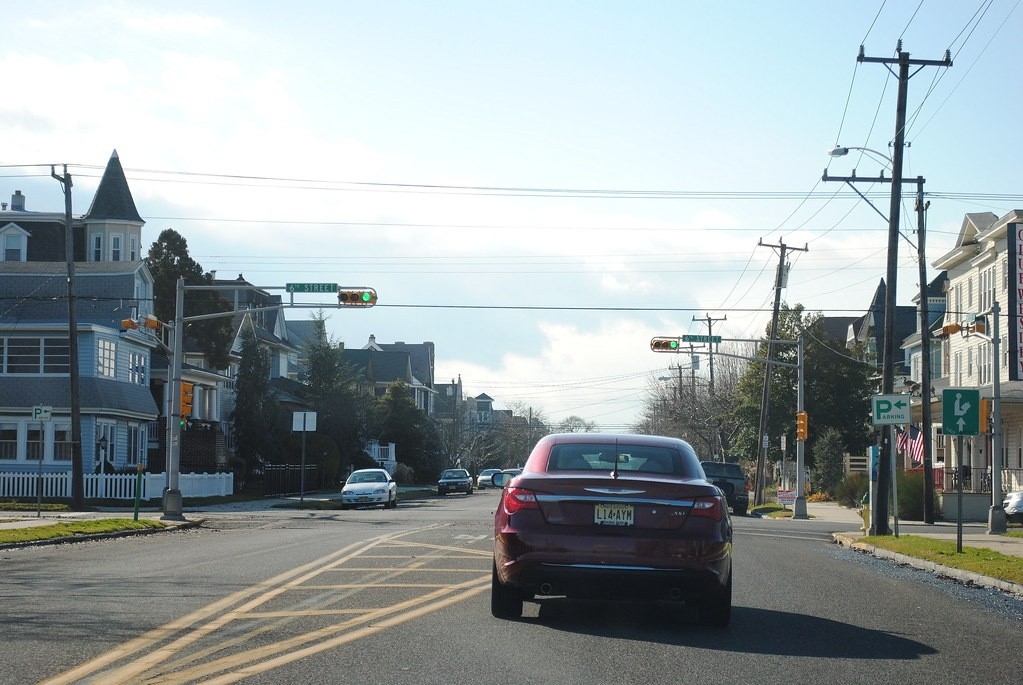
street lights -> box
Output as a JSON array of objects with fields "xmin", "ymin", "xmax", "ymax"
[{"xmin": 829, "ymin": 146, "xmax": 903, "ymax": 534}]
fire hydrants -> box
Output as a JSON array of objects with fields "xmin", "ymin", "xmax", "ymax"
[{"xmin": 855, "ymin": 492, "xmax": 871, "ymax": 538}]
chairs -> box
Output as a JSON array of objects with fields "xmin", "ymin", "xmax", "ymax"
[
  {"xmin": 376, "ymin": 475, "xmax": 383, "ymax": 481},
  {"xmin": 639, "ymin": 459, "xmax": 670, "ymax": 474},
  {"xmin": 448, "ymin": 475, "xmax": 452, "ymax": 477},
  {"xmin": 952, "ymin": 466, "xmax": 970, "ymax": 489},
  {"xmin": 358, "ymin": 478, "xmax": 364, "ymax": 482},
  {"xmin": 567, "ymin": 458, "xmax": 591, "ymax": 470}
]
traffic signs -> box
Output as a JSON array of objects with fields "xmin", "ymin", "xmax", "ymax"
[
  {"xmin": 285, "ymin": 283, "xmax": 338, "ymax": 292},
  {"xmin": 684, "ymin": 334, "xmax": 721, "ymax": 342}
]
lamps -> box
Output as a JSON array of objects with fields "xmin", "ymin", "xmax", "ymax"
[{"xmin": 98, "ymin": 436, "xmax": 107, "ymax": 449}]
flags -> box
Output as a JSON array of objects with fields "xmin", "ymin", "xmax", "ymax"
[
  {"xmin": 908, "ymin": 425, "xmax": 923, "ymax": 462},
  {"xmin": 895, "ymin": 426, "xmax": 909, "ymax": 450}
]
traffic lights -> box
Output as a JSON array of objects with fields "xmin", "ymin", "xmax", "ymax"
[
  {"xmin": 121, "ymin": 318, "xmax": 137, "ymax": 329},
  {"xmin": 650, "ymin": 339, "xmax": 678, "ymax": 350},
  {"xmin": 942, "ymin": 323, "xmax": 959, "ymax": 336},
  {"xmin": 337, "ymin": 291, "xmax": 375, "ymax": 304},
  {"xmin": 181, "ymin": 381, "xmax": 194, "ymax": 415},
  {"xmin": 181, "ymin": 418, "xmax": 187, "ymax": 427}
]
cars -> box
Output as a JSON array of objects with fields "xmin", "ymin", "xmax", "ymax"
[
  {"xmin": 437, "ymin": 468, "xmax": 473, "ymax": 495},
  {"xmin": 476, "ymin": 470, "xmax": 521, "ymax": 490},
  {"xmin": 341, "ymin": 469, "xmax": 399, "ymax": 509},
  {"xmin": 491, "ymin": 433, "xmax": 733, "ymax": 622},
  {"xmin": 1003, "ymin": 490, "xmax": 1023, "ymax": 526}
]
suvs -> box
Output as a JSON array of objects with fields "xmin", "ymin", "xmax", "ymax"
[{"xmin": 699, "ymin": 461, "xmax": 749, "ymax": 514}]
